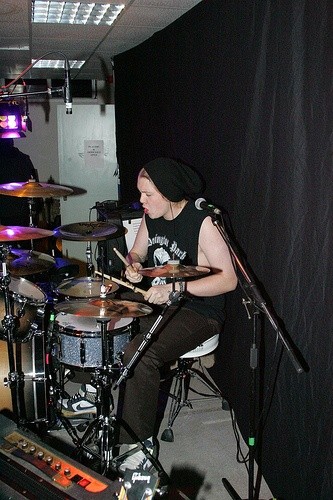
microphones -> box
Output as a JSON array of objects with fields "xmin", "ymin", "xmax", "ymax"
[
  {"xmin": 194, "ymin": 198, "xmax": 223, "ymax": 215},
  {"xmin": 64, "ymin": 60, "xmax": 73, "ymax": 114}
]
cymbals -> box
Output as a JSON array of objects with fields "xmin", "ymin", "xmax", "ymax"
[
  {"xmin": 0, "ymin": 181, "xmax": 74, "ymax": 198},
  {"xmin": 54, "ymin": 221, "xmax": 129, "ymax": 242},
  {"xmin": 0, "ymin": 225, "xmax": 56, "ymax": 242},
  {"xmin": 137, "ymin": 264, "xmax": 210, "ymax": 278},
  {"xmin": 53, "ymin": 299, "xmax": 153, "ymax": 319},
  {"xmin": 0, "ymin": 249, "xmax": 56, "ymax": 275}
]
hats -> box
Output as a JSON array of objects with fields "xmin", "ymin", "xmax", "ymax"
[{"xmin": 143, "ymin": 158, "xmax": 203, "ymax": 202}]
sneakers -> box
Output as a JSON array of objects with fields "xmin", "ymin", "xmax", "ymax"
[
  {"xmin": 120, "ymin": 437, "xmax": 154, "ymax": 472},
  {"xmin": 56, "ymin": 384, "xmax": 113, "ymax": 416}
]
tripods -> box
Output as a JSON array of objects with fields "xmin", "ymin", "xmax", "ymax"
[{"xmin": 67, "ymin": 318, "xmax": 175, "ymax": 500}]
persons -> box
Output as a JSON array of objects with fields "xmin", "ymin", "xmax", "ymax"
[
  {"xmin": 113, "ymin": 152, "xmax": 237, "ymax": 474},
  {"xmin": 0, "ymin": 139, "xmax": 44, "ymax": 250}
]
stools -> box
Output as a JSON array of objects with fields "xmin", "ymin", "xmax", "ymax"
[{"xmin": 160, "ymin": 334, "xmax": 233, "ymax": 442}]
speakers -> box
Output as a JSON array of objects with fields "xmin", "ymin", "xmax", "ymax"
[{"xmin": 96, "ymin": 209, "xmax": 144, "ymax": 279}]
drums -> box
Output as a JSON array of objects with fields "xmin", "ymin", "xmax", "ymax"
[
  {"xmin": 0, "ymin": 273, "xmax": 49, "ymax": 343},
  {"xmin": 54, "ymin": 277, "xmax": 120, "ymax": 304},
  {"xmin": 50, "ymin": 310, "xmax": 139, "ymax": 373},
  {"xmin": 0, "ymin": 307, "xmax": 58, "ymax": 432}
]
